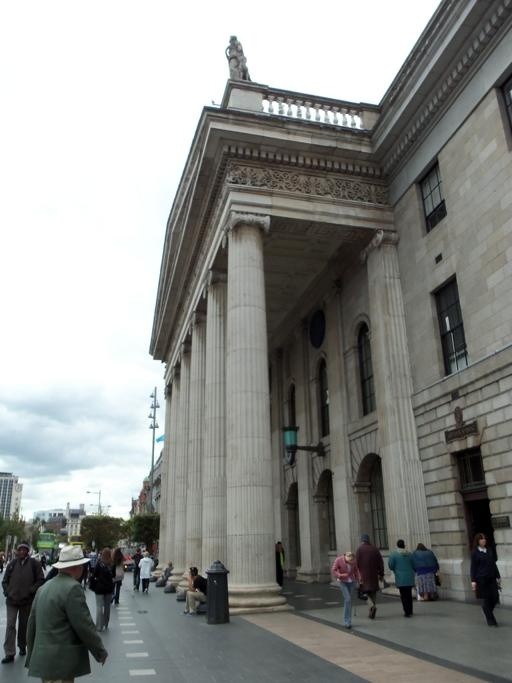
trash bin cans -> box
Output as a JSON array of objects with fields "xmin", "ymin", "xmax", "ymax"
[{"xmin": 204, "ymin": 560, "xmax": 231, "ymax": 625}]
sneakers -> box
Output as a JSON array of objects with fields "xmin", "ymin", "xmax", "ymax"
[
  {"xmin": 182, "ymin": 609, "xmax": 196, "ymax": 616},
  {"xmin": 19, "ymin": 648, "xmax": 26, "ymax": 656},
  {"xmin": 1, "ymin": 656, "xmax": 15, "ymax": 664},
  {"xmin": 370, "ymin": 607, "xmax": 376, "ymax": 618},
  {"xmin": 345, "ymin": 623, "xmax": 352, "ymax": 629},
  {"xmin": 110, "ymin": 586, "xmax": 148, "ymax": 605},
  {"xmin": 95, "ymin": 625, "xmax": 109, "ymax": 632}
]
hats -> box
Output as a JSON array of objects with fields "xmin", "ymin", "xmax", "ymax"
[
  {"xmin": 51, "ymin": 544, "xmax": 92, "ymax": 570},
  {"xmin": 16, "ymin": 541, "xmax": 30, "ymax": 552},
  {"xmin": 361, "ymin": 534, "xmax": 370, "ymax": 543}
]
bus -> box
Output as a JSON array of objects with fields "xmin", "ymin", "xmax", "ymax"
[{"xmin": 30, "ymin": 531, "xmax": 158, "ymax": 574}]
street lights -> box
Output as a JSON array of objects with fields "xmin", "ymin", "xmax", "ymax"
[
  {"xmin": 86, "ymin": 488, "xmax": 112, "ymax": 553},
  {"xmin": 149, "ymin": 385, "xmax": 160, "ymax": 514}
]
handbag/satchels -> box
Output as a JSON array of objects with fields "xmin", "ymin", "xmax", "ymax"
[{"xmin": 434, "ymin": 573, "xmax": 441, "ymax": 586}]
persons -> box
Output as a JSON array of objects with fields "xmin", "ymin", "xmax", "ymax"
[
  {"xmin": 0, "ymin": 537, "xmax": 160, "ymax": 633},
  {"xmin": 410, "ymin": 540, "xmax": 440, "ymax": 601},
  {"xmin": 276, "ymin": 541, "xmax": 287, "ymax": 589},
  {"xmin": 330, "ymin": 550, "xmax": 365, "ymax": 630},
  {"xmin": 1, "ymin": 540, "xmax": 47, "ymax": 662},
  {"xmin": 388, "ymin": 537, "xmax": 417, "ymax": 617},
  {"xmin": 183, "ymin": 565, "xmax": 208, "ymax": 617},
  {"xmin": 24, "ymin": 544, "xmax": 109, "ymax": 682},
  {"xmin": 353, "ymin": 531, "xmax": 386, "ymax": 619},
  {"xmin": 468, "ymin": 532, "xmax": 503, "ymax": 629}
]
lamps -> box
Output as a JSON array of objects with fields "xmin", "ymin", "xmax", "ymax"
[{"xmin": 281, "ymin": 423, "xmax": 326, "ymax": 466}]
